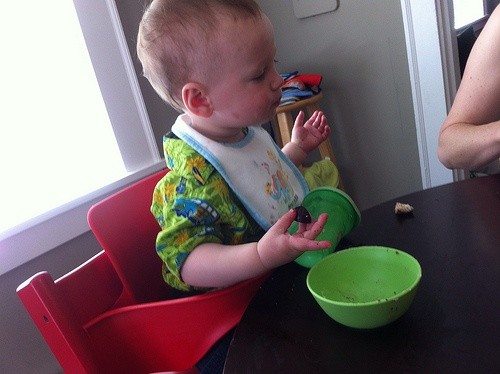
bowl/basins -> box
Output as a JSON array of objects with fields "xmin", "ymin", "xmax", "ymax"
[{"xmin": 306, "ymin": 246, "xmax": 423, "ymax": 328}]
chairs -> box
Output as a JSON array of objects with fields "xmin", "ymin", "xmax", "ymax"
[{"xmin": 12, "ymin": 168, "xmax": 254, "ymax": 374}]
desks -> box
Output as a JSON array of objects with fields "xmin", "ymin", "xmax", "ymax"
[{"xmin": 222, "ymin": 172, "xmax": 499, "ymax": 373}]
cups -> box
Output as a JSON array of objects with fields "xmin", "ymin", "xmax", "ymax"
[{"xmin": 287, "ymin": 187, "xmax": 362, "ymax": 268}]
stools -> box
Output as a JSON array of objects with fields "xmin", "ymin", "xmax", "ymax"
[{"xmin": 269, "ymin": 88, "xmax": 344, "ymax": 193}]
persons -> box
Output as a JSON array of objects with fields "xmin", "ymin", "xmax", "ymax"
[
  {"xmin": 136, "ymin": 0, "xmax": 331, "ymax": 292},
  {"xmin": 437, "ymin": 0, "xmax": 500, "ymax": 179}
]
